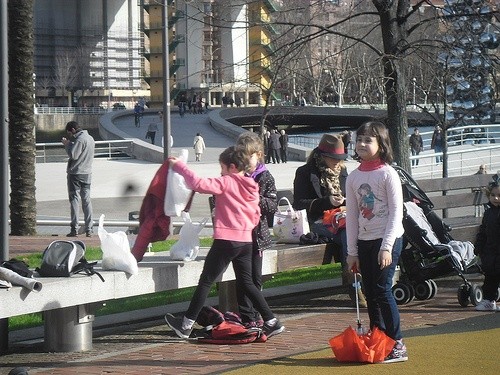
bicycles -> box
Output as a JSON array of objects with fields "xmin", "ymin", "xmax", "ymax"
[
  {"xmin": 135, "ymin": 112, "xmax": 140, "ymax": 129},
  {"xmin": 178, "ymin": 101, "xmax": 187, "ymax": 118}
]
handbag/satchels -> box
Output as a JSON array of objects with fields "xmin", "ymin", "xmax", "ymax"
[
  {"xmin": 273, "ymin": 197, "xmax": 309, "ymax": 244},
  {"xmin": 171, "ymin": 211, "xmax": 209, "ymax": 263}
]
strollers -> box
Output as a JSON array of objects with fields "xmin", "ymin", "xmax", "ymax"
[{"xmin": 388, "ymin": 165, "xmax": 486, "ymax": 308}]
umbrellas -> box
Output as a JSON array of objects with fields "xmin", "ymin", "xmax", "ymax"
[{"xmin": 327, "ymin": 266, "xmax": 396, "ymax": 363}]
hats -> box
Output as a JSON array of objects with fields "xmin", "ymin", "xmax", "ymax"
[{"xmin": 314, "ymin": 134, "xmax": 349, "ymax": 160}]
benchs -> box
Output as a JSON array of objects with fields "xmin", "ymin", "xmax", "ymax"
[
  {"xmin": 0, "ymin": 247, "xmax": 278, "ymax": 352},
  {"xmin": 207, "ymin": 174, "xmax": 499, "ymax": 272}
]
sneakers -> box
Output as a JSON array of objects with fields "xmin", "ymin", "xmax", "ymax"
[
  {"xmin": 165, "ymin": 312, "xmax": 194, "ymax": 339},
  {"xmin": 476, "ymin": 299, "xmax": 500, "ymax": 311},
  {"xmin": 262, "ymin": 319, "xmax": 286, "ymax": 339}
]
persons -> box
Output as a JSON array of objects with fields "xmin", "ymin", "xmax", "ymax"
[
  {"xmin": 295, "ymin": 97, "xmax": 306, "ymax": 106},
  {"xmin": 431, "ymin": 125, "xmax": 444, "ymax": 165},
  {"xmin": 474, "ymin": 180, "xmax": 500, "ymax": 311},
  {"xmin": 164, "ymin": 145, "xmax": 284, "ymax": 340},
  {"xmin": 134, "ymin": 100, "xmax": 144, "ymax": 121},
  {"xmin": 62, "ymin": 121, "xmax": 93, "ymax": 238},
  {"xmin": 472, "ymin": 164, "xmax": 489, "ymax": 210},
  {"xmin": 148, "ymin": 118, "xmax": 159, "ymax": 144},
  {"xmin": 292, "ymin": 135, "xmax": 366, "ymax": 308},
  {"xmin": 236, "ymin": 131, "xmax": 277, "ymax": 329},
  {"xmin": 194, "ymin": 133, "xmax": 205, "ymax": 161},
  {"xmin": 265, "ymin": 130, "xmax": 288, "ymax": 164},
  {"xmin": 222, "ymin": 94, "xmax": 241, "ymax": 108},
  {"xmin": 342, "ymin": 130, "xmax": 351, "ymax": 154},
  {"xmin": 178, "ymin": 93, "xmax": 208, "ymax": 115},
  {"xmin": 346, "ymin": 122, "xmax": 407, "ymax": 364},
  {"xmin": 409, "ymin": 129, "xmax": 424, "ymax": 168}
]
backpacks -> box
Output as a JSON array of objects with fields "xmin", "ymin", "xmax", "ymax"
[{"xmin": 36, "ymin": 240, "xmax": 98, "ymax": 277}]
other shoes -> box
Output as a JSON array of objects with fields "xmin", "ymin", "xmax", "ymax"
[
  {"xmin": 67, "ymin": 231, "xmax": 79, "ymax": 237},
  {"xmin": 246, "ymin": 320, "xmax": 264, "ymax": 327},
  {"xmin": 382, "ymin": 347, "xmax": 409, "ymax": 364}
]
